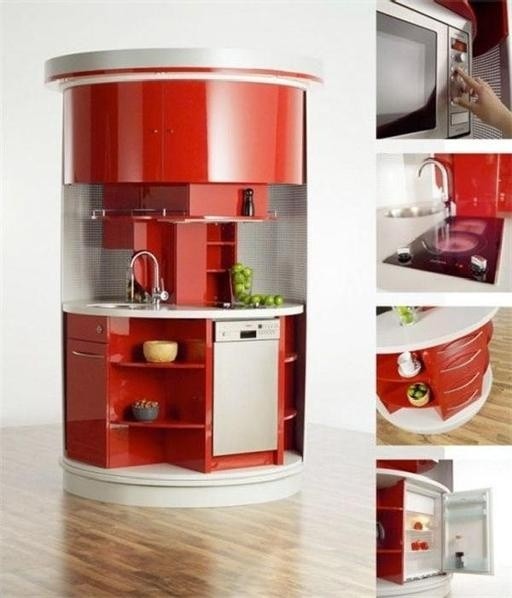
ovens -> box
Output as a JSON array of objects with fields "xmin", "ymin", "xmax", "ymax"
[{"xmin": 214, "ymin": 319, "xmax": 281, "ymax": 456}]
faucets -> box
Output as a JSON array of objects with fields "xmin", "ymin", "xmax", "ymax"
[
  {"xmin": 418, "ymin": 160, "xmax": 452, "ymax": 220},
  {"xmin": 130, "ymin": 250, "xmax": 167, "ymax": 303},
  {"xmin": 421, "ymin": 238, "xmax": 441, "ymax": 256}
]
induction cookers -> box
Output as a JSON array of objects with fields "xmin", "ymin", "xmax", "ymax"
[{"xmin": 381, "ymin": 214, "xmax": 505, "ymax": 285}]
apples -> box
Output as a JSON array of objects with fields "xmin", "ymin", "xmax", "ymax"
[
  {"xmin": 420, "ymin": 542, "xmax": 428, "ymax": 549},
  {"xmin": 411, "ymin": 542, "xmax": 419, "ymax": 550},
  {"xmin": 415, "ymin": 522, "xmax": 423, "ymax": 529}
]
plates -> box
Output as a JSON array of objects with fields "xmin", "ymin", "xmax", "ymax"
[{"xmin": 398, "ymin": 361, "xmax": 421, "ymax": 378}]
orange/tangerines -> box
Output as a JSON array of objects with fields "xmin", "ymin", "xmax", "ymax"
[
  {"xmin": 232, "ymin": 263, "xmax": 284, "ymax": 306},
  {"xmin": 398, "ymin": 306, "xmax": 413, "ymax": 324},
  {"xmin": 408, "ymin": 383, "xmax": 426, "ymax": 399}
]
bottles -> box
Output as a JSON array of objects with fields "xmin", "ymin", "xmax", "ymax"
[
  {"xmin": 453, "ymin": 534, "xmax": 467, "ymax": 568},
  {"xmin": 243, "ymin": 187, "xmax": 255, "ymax": 217}
]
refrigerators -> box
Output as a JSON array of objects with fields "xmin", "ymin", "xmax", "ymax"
[{"xmin": 402, "ymin": 481, "xmax": 493, "ymax": 582}]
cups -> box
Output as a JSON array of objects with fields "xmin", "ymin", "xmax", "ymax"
[
  {"xmin": 397, "ymin": 351, "xmax": 418, "ymax": 374},
  {"xmin": 392, "ymin": 306, "xmax": 418, "ymax": 327},
  {"xmin": 229, "ymin": 269, "xmax": 253, "ymax": 307}
]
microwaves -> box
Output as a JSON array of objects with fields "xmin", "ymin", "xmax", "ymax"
[{"xmin": 376, "ymin": 0, "xmax": 474, "ymax": 139}]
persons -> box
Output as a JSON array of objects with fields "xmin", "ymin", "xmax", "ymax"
[{"xmin": 452, "ymin": 66, "xmax": 512, "ymax": 139}]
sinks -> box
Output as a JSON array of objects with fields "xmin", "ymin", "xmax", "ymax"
[
  {"xmin": 88, "ymin": 302, "xmax": 134, "ymax": 308},
  {"xmin": 432, "ymin": 230, "xmax": 478, "ymax": 253}
]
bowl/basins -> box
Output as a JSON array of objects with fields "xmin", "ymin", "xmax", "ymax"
[
  {"xmin": 407, "ymin": 383, "xmax": 431, "ymax": 406},
  {"xmin": 132, "ymin": 406, "xmax": 159, "ymax": 423},
  {"xmin": 143, "ymin": 341, "xmax": 179, "ymax": 363}
]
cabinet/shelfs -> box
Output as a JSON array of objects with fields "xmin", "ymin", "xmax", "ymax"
[
  {"xmin": 277, "ymin": 315, "xmax": 298, "ymax": 466},
  {"xmin": 376, "ymin": 321, "xmax": 494, "ymax": 421},
  {"xmin": 119, "ymin": 80, "xmax": 208, "ymax": 183},
  {"xmin": 176, "ymin": 221, "xmax": 237, "ymax": 306},
  {"xmin": 377, "ymin": 505, "xmax": 403, "ymax": 554},
  {"xmin": 64, "ymin": 314, "xmax": 211, "ymax": 473}
]
